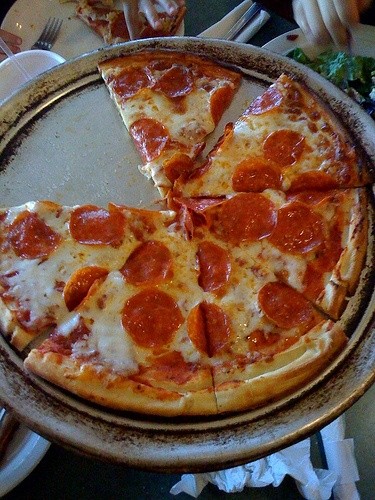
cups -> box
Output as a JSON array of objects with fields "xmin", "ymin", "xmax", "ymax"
[{"xmin": 0, "ymin": 50, "xmax": 64, "ymax": 105}]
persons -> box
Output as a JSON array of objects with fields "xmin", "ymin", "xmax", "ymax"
[
  {"xmin": 252, "ymin": 0, "xmax": 360, "ymax": 56},
  {"xmin": 124, "ymin": 0, "xmax": 186, "ymax": 41},
  {"xmin": 0, "ymin": 31, "xmax": 23, "ymax": 62}
]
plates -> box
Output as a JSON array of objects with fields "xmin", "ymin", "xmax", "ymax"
[
  {"xmin": 0, "ymin": 37, "xmax": 375, "ymax": 472},
  {"xmin": 0, "ymin": 408, "xmax": 51, "ymax": 498}
]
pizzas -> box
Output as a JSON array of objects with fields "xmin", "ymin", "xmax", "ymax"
[{"xmin": 0, "ymin": 49, "xmax": 371, "ymax": 417}]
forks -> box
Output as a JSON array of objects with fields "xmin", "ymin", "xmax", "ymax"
[{"xmin": 30, "ymin": 15, "xmax": 66, "ymax": 52}]
